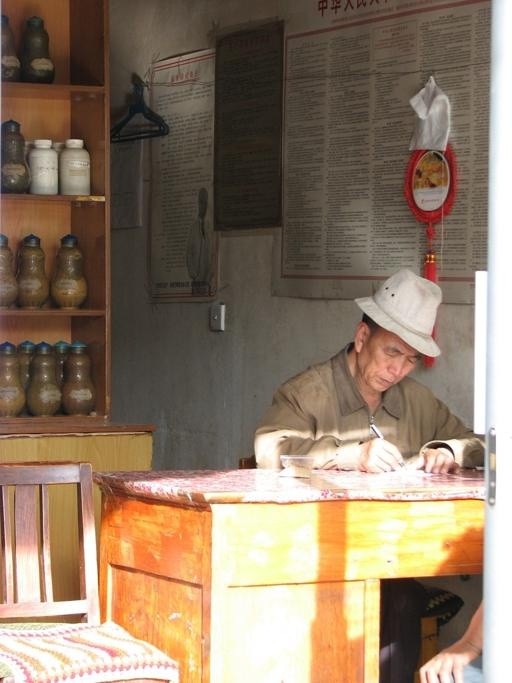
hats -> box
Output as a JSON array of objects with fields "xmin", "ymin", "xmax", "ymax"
[{"xmin": 352, "ymin": 264, "xmax": 446, "ymax": 359}]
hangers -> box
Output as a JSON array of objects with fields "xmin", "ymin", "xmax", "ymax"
[{"xmin": 110, "ymin": 74, "xmax": 170, "ymax": 144}]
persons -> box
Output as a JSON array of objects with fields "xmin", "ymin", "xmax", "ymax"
[
  {"xmin": 254, "ymin": 269, "xmax": 485, "ymax": 683},
  {"xmin": 419, "ymin": 599, "xmax": 484, "ymax": 683},
  {"xmin": 186, "ymin": 188, "xmax": 216, "ymax": 297}
]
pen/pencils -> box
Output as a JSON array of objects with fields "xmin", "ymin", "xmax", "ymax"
[{"xmin": 369, "ymin": 423, "xmax": 407, "ymax": 471}]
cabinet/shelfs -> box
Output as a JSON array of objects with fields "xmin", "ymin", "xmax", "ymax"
[{"xmin": 1, "ymin": 1, "xmax": 113, "ymax": 426}]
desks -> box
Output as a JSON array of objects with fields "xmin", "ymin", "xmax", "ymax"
[{"xmin": 98, "ymin": 469, "xmax": 485, "ymax": 682}]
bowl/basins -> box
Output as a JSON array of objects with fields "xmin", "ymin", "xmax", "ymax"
[{"xmin": 280, "ymin": 455, "xmax": 316, "ymax": 472}]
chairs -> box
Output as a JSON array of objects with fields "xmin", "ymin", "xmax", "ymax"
[
  {"xmin": 0, "ymin": 461, "xmax": 179, "ymax": 683},
  {"xmin": 238, "ymin": 455, "xmax": 439, "ymax": 681}
]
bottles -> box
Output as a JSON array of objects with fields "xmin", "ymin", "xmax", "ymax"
[
  {"xmin": 0, "ymin": 15, "xmax": 50, "ymax": 82},
  {"xmin": 0, "ymin": 234, "xmax": 89, "ymax": 311},
  {"xmin": 1, "ymin": 119, "xmax": 90, "ymax": 197},
  {"xmin": 0, "ymin": 341, "xmax": 97, "ymax": 417}
]
